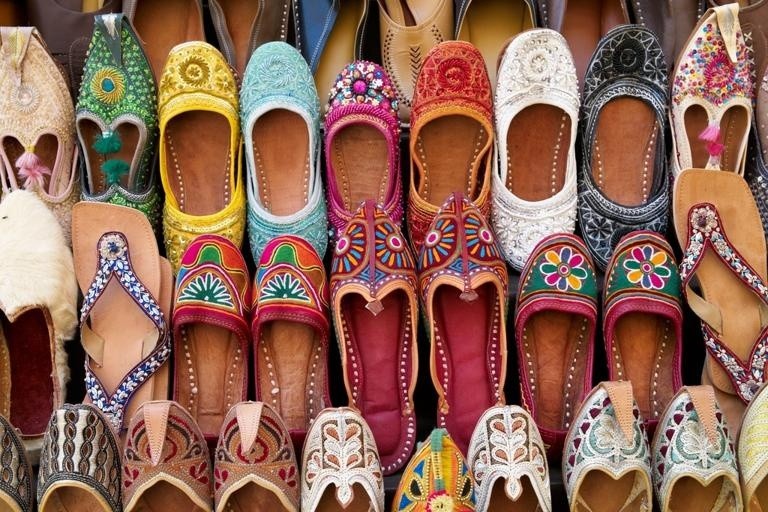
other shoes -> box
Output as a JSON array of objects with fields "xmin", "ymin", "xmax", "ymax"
[{"xmin": 1, "ymin": 1, "xmax": 764, "ymax": 512}]
